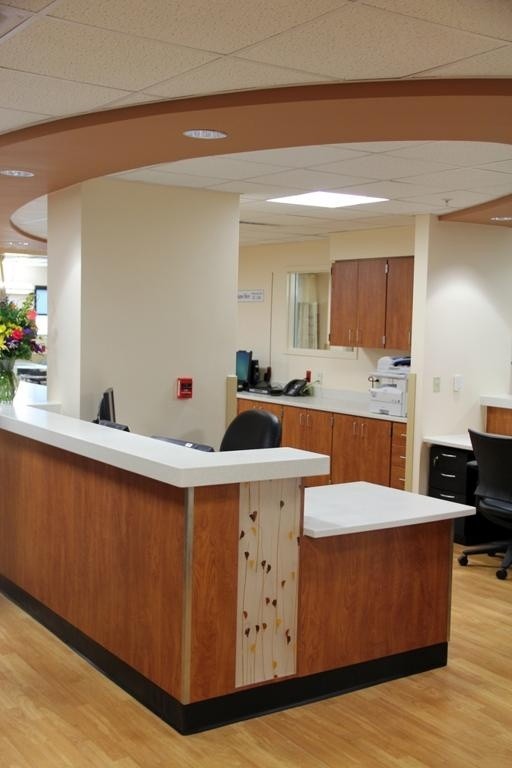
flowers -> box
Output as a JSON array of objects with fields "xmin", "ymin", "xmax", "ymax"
[{"xmin": 0, "ymin": 291, "xmax": 46, "ymax": 402}]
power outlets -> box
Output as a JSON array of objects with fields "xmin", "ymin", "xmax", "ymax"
[{"xmin": 316, "ymin": 372, "xmax": 323, "ymax": 385}]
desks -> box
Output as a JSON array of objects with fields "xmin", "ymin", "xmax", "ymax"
[{"xmin": 423, "ymin": 433, "xmax": 476, "ymax": 546}]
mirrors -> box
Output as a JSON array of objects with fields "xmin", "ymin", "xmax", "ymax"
[{"xmin": 282, "ymin": 266, "xmax": 358, "ymax": 360}]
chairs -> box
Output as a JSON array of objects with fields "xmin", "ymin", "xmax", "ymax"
[
  {"xmin": 220, "ymin": 408, "xmax": 281, "ymax": 451},
  {"xmin": 457, "ymin": 429, "xmax": 512, "ymax": 579}
]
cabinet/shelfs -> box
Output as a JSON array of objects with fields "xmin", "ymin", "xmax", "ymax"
[
  {"xmin": 330, "ymin": 412, "xmax": 392, "ymax": 487},
  {"xmin": 282, "ymin": 404, "xmax": 333, "ymax": 487},
  {"xmin": 328, "ymin": 258, "xmax": 384, "ymax": 348},
  {"xmin": 385, "ymin": 255, "xmax": 414, "ymax": 350},
  {"xmin": 237, "ymin": 398, "xmax": 283, "ymax": 421},
  {"xmin": 390, "ymin": 421, "xmax": 407, "ymax": 491}
]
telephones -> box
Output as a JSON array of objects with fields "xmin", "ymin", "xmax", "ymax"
[{"xmin": 282, "ymin": 379, "xmax": 307, "ymax": 396}]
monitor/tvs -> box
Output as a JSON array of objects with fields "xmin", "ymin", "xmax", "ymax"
[
  {"xmin": 236, "ymin": 350, "xmax": 252, "ymax": 384},
  {"xmin": 98, "ymin": 387, "xmax": 115, "ymax": 423}
]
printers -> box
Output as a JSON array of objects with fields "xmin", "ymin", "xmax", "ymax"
[{"xmin": 367, "ymin": 356, "xmax": 411, "ymax": 418}]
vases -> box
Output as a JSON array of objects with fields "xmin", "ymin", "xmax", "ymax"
[{"xmin": 0, "ymin": 359, "xmax": 20, "ymax": 406}]
